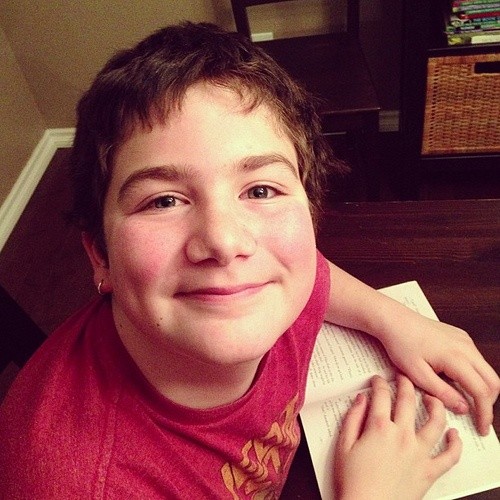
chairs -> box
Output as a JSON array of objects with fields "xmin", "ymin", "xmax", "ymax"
[{"xmin": 228, "ymin": 0, "xmax": 383, "ymax": 170}]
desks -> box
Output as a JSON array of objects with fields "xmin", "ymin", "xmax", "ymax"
[{"xmin": 280, "ymin": 199, "xmax": 500, "ymax": 496}]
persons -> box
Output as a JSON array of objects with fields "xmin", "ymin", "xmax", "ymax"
[{"xmin": 0, "ymin": 20, "xmax": 500, "ymax": 500}]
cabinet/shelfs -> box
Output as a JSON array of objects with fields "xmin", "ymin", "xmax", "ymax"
[{"xmin": 400, "ymin": 0, "xmax": 499, "ymax": 165}]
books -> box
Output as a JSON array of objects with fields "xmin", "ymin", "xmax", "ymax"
[
  {"xmin": 447, "ymin": 0, "xmax": 500, "ymax": 45},
  {"xmin": 299, "ymin": 281, "xmax": 500, "ymax": 500}
]
